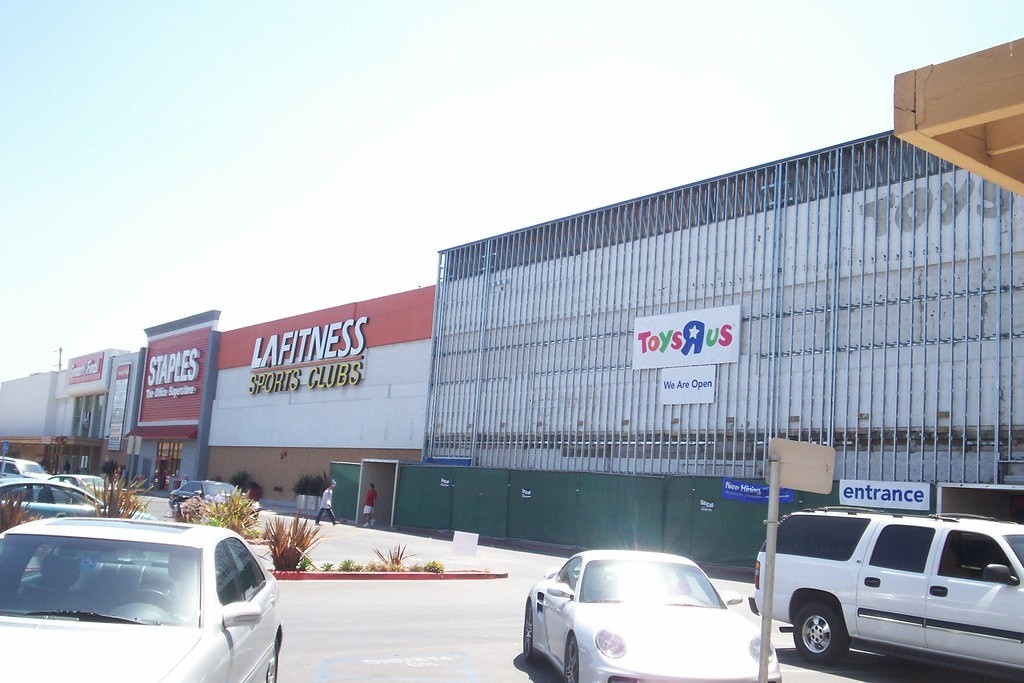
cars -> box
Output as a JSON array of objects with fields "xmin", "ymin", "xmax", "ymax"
[
  {"xmin": 0, "ymin": 515, "xmax": 282, "ymax": 683},
  {"xmin": 169, "ymin": 481, "xmax": 261, "ymax": 520},
  {"xmin": 523, "ymin": 550, "xmax": 782, "ymax": 683},
  {"xmin": 0, "ymin": 456, "xmax": 159, "ymax": 520}
]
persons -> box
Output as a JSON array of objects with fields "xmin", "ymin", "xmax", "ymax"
[
  {"xmin": 315, "ymin": 483, "xmax": 340, "ymax": 525},
  {"xmin": 363, "ymin": 483, "xmax": 377, "ymax": 527}
]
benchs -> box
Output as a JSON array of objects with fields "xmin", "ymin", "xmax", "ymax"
[{"xmin": 74, "ymin": 563, "xmax": 170, "ymax": 612}]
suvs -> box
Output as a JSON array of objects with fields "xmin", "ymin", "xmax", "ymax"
[{"xmin": 748, "ymin": 506, "xmax": 1024, "ymax": 682}]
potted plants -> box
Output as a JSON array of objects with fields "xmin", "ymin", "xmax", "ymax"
[{"xmin": 291, "ymin": 469, "xmax": 330, "ymax": 511}]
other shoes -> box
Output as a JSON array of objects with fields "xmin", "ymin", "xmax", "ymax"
[
  {"xmin": 363, "ymin": 523, "xmax": 369, "ymax": 527},
  {"xmin": 333, "ymin": 521, "xmax": 339, "ymax": 526},
  {"xmin": 371, "ymin": 519, "xmax": 375, "ymax": 525},
  {"xmin": 315, "ymin": 522, "xmax": 320, "ymax": 525}
]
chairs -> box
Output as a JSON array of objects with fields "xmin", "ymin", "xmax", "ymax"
[
  {"xmin": 38, "ymin": 489, "xmax": 49, "ymax": 502},
  {"xmin": 20, "ymin": 555, "xmax": 83, "ymax": 611},
  {"xmin": 169, "ymin": 554, "xmax": 198, "ymax": 580}
]
